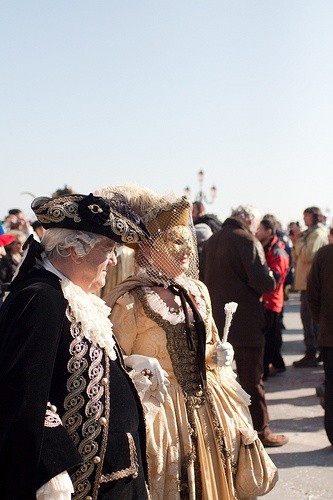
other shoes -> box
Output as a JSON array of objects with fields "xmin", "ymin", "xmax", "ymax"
[
  {"xmin": 258, "ymin": 426, "xmax": 289, "ymax": 447},
  {"xmin": 316, "ymin": 376, "xmax": 326, "ymax": 409},
  {"xmin": 268, "ymin": 361, "xmax": 286, "ymax": 375},
  {"xmin": 293, "ymin": 355, "xmax": 318, "ymax": 367}
]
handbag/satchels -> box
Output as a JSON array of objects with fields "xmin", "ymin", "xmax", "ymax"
[{"xmin": 236, "ymin": 426, "xmax": 279, "ymax": 498}]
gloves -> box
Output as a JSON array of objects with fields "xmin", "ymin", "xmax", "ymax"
[
  {"xmin": 211, "ymin": 341, "xmax": 234, "ymax": 367},
  {"xmin": 137, "ymin": 370, "xmax": 170, "ymax": 407},
  {"xmin": 36, "ymin": 470, "xmax": 75, "ymax": 500},
  {"xmin": 123, "ymin": 354, "xmax": 170, "ymax": 391}
]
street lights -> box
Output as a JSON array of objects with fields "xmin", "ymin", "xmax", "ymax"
[{"xmin": 185, "ymin": 169, "xmax": 217, "ymax": 209}]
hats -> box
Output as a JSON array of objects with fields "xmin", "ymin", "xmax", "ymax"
[
  {"xmin": 31, "ymin": 193, "xmax": 151, "ymax": 250},
  {"xmin": 194, "ymin": 223, "xmax": 213, "ymax": 242},
  {"xmin": 148, "ymin": 202, "xmax": 190, "ymax": 241},
  {"xmin": 0, "ymin": 222, "xmax": 22, "ymax": 246}
]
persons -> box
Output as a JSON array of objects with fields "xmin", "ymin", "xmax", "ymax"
[
  {"xmin": 0, "ymin": 193, "xmax": 169, "ymax": 500},
  {"xmin": 94, "ymin": 186, "xmax": 279, "ymax": 500},
  {"xmin": 0, "ymin": 201, "xmax": 333, "ymax": 447}
]
